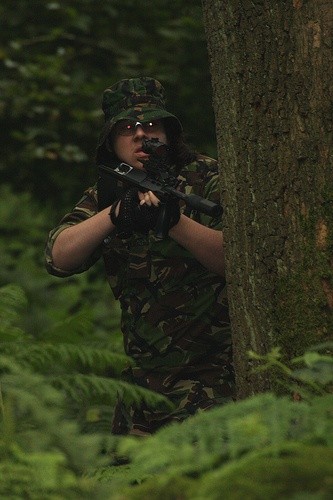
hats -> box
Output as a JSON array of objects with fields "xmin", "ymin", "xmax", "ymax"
[{"xmin": 96, "ymin": 78, "xmax": 184, "ymax": 149}]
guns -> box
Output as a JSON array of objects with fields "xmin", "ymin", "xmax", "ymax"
[{"xmin": 95, "ymin": 137, "xmax": 223, "ymax": 234}]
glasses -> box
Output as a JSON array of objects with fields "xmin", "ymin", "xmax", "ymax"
[{"xmin": 113, "ymin": 119, "xmax": 160, "ymax": 136}]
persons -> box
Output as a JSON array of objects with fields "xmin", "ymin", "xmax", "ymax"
[{"xmin": 43, "ymin": 75, "xmax": 237, "ymax": 465}]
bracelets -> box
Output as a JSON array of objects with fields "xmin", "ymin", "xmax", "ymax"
[{"xmin": 108, "ymin": 201, "xmax": 124, "ymax": 229}]
